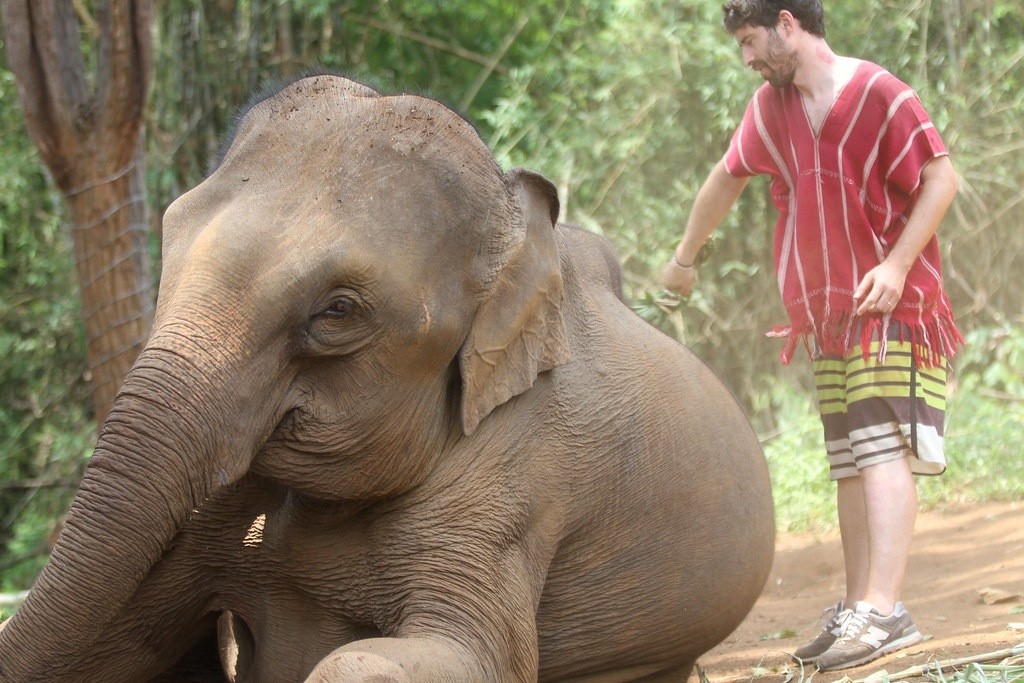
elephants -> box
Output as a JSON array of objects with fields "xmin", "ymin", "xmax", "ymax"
[{"xmin": 0, "ymin": 76, "xmax": 774, "ymax": 683}]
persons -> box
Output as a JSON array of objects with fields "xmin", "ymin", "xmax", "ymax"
[{"xmin": 658, "ymin": 0, "xmax": 965, "ymax": 673}]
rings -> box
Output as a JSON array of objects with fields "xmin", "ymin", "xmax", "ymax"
[{"xmin": 887, "ymin": 302, "xmax": 893, "ymax": 306}]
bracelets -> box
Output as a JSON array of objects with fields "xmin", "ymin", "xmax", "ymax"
[{"xmin": 673, "ymin": 255, "xmax": 694, "ymax": 270}]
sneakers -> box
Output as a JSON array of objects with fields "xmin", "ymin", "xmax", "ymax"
[
  {"xmin": 814, "ymin": 600, "xmax": 924, "ymax": 672},
  {"xmin": 791, "ymin": 600, "xmax": 858, "ymax": 664}
]
